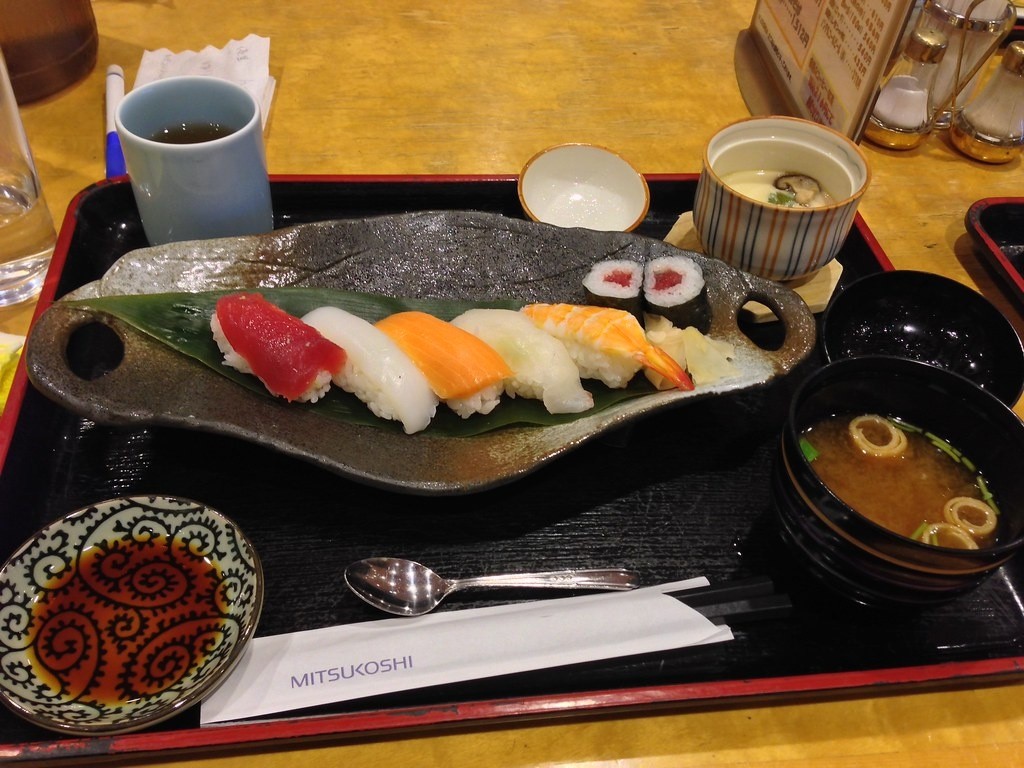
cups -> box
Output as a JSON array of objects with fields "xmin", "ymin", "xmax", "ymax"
[
  {"xmin": 0, "ymin": 0, "xmax": 99, "ymax": 106},
  {"xmin": 114, "ymin": 75, "xmax": 275, "ymax": 247},
  {"xmin": 0, "ymin": 49, "xmax": 58, "ymax": 307},
  {"xmin": 693, "ymin": 115, "xmax": 872, "ymax": 290}
]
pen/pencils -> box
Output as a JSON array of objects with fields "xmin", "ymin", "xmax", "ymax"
[{"xmin": 104, "ymin": 63, "xmax": 126, "ymax": 179}]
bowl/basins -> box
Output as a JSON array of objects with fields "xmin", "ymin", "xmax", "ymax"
[
  {"xmin": 773, "ymin": 355, "xmax": 1024, "ymax": 617},
  {"xmin": 822, "ymin": 270, "xmax": 1024, "ymax": 410}
]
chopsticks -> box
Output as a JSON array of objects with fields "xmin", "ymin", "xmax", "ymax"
[{"xmin": 663, "ymin": 575, "xmax": 792, "ymax": 619}]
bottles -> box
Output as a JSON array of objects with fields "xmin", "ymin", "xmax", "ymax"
[
  {"xmin": 863, "ymin": 0, "xmax": 1016, "ymax": 130},
  {"xmin": 865, "ymin": 27, "xmax": 950, "ymax": 151},
  {"xmin": 950, "ymin": 41, "xmax": 1024, "ymax": 165}
]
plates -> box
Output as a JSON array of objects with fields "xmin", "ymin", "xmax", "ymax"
[
  {"xmin": 26, "ymin": 210, "xmax": 816, "ymax": 499},
  {"xmin": 0, "ymin": 493, "xmax": 264, "ymax": 737},
  {"xmin": 517, "ymin": 143, "xmax": 651, "ymax": 233}
]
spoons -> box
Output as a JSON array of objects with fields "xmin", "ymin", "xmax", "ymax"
[{"xmin": 343, "ymin": 557, "xmax": 638, "ymax": 617}]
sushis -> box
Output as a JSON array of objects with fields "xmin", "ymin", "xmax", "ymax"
[
  {"xmin": 449, "ymin": 310, "xmax": 594, "ymax": 414},
  {"xmin": 521, "ymin": 304, "xmax": 693, "ymax": 393},
  {"xmin": 583, "ymin": 259, "xmax": 645, "ymax": 327},
  {"xmin": 212, "ymin": 295, "xmax": 349, "ymax": 404},
  {"xmin": 300, "ymin": 308, "xmax": 439, "ymax": 434},
  {"xmin": 641, "ymin": 257, "xmax": 711, "ymax": 334},
  {"xmin": 373, "ymin": 311, "xmax": 515, "ymax": 419}
]
